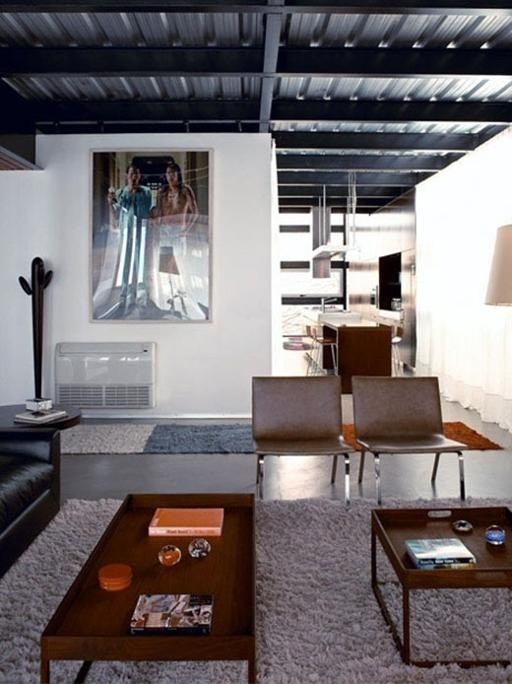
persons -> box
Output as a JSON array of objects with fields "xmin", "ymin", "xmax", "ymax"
[
  {"xmin": 150, "ymin": 161, "xmax": 200, "ymax": 309},
  {"xmin": 106, "ymin": 166, "xmax": 153, "ymax": 311}
]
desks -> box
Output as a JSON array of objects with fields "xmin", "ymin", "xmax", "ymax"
[{"xmin": 316, "ymin": 313, "xmax": 392, "ymax": 377}]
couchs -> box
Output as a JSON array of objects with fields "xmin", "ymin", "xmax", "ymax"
[{"xmin": 0, "ymin": 429, "xmax": 62, "ymax": 579}]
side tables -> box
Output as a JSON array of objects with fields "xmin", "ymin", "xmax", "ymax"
[{"xmin": 0, "ymin": 397, "xmax": 82, "ymax": 427}]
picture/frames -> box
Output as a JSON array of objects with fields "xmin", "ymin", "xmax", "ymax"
[{"xmin": 90, "ymin": 150, "xmax": 212, "ymax": 322}]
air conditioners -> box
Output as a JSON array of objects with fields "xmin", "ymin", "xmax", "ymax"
[{"xmin": 54, "ymin": 342, "xmax": 154, "ymax": 410}]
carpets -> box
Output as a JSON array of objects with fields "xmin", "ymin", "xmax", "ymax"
[
  {"xmin": 58, "ymin": 417, "xmax": 156, "ymax": 453},
  {"xmin": 0, "ymin": 498, "xmax": 512, "ymax": 684},
  {"xmin": 340, "ymin": 422, "xmax": 507, "ymax": 452},
  {"xmin": 142, "ymin": 421, "xmax": 256, "ymax": 455}
]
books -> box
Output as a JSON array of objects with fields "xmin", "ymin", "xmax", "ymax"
[
  {"xmin": 129, "ymin": 593, "xmax": 215, "ymax": 635},
  {"xmin": 12, "ymin": 409, "xmax": 68, "ymax": 424},
  {"xmin": 402, "ymin": 538, "xmax": 477, "ymax": 571},
  {"xmin": 147, "ymin": 507, "xmax": 225, "ymax": 537}
]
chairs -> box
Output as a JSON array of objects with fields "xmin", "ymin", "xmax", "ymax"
[
  {"xmin": 351, "ymin": 376, "xmax": 468, "ymax": 505},
  {"xmin": 304, "ymin": 325, "xmax": 323, "ymax": 377},
  {"xmin": 392, "ymin": 327, "xmax": 403, "ymax": 371},
  {"xmin": 305, "ymin": 329, "xmax": 338, "ymax": 376},
  {"xmin": 251, "ymin": 376, "xmax": 349, "ymax": 506}
]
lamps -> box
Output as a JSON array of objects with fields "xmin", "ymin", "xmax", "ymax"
[{"xmin": 485, "ymin": 224, "xmax": 512, "ymax": 306}]
